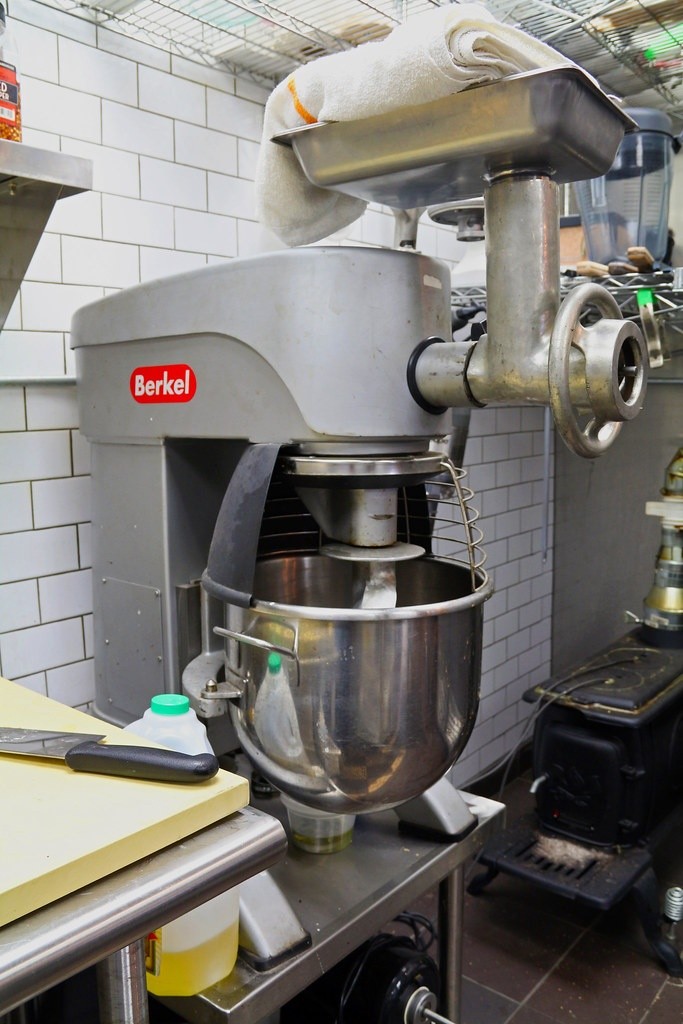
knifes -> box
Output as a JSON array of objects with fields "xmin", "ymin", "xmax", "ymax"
[{"xmin": 1, "ymin": 726, "xmax": 218, "ymax": 783}]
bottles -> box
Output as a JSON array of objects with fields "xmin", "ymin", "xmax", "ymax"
[
  {"xmin": 251, "ymin": 653, "xmax": 309, "ymax": 774},
  {"xmin": 99, "ymin": 694, "xmax": 240, "ymax": 997}
]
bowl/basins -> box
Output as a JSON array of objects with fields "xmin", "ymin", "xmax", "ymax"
[{"xmin": 280, "ymin": 792, "xmax": 356, "ymax": 854}]
